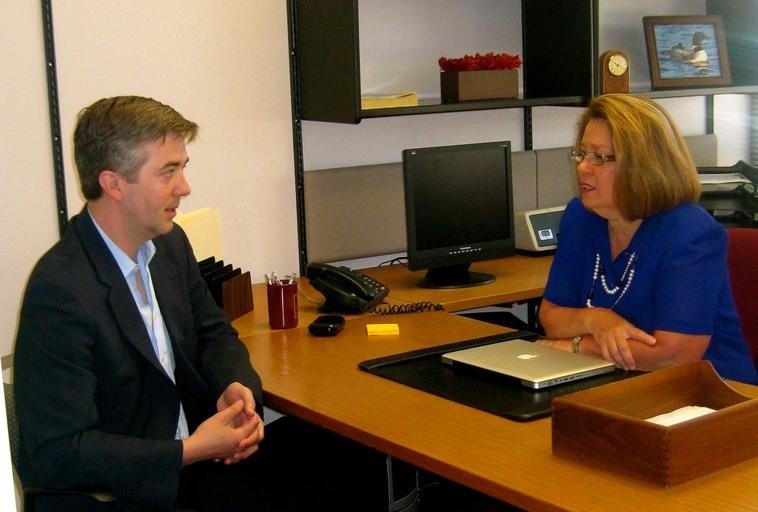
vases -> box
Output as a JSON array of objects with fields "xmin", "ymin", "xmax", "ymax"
[{"xmin": 440, "ymin": 70, "xmax": 520, "ymax": 103}]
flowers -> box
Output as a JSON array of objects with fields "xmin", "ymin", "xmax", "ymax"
[{"xmin": 439, "ymin": 51, "xmax": 525, "ymax": 76}]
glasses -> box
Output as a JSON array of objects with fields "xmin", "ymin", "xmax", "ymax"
[{"xmin": 571, "ymin": 150, "xmax": 616, "ymax": 166}]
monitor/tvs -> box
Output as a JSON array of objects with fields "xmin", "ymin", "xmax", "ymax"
[{"xmin": 402, "ymin": 140, "xmax": 515, "ymax": 289}]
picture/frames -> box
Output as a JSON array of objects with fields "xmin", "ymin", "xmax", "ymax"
[{"xmin": 639, "ymin": 11, "xmax": 733, "ymax": 90}]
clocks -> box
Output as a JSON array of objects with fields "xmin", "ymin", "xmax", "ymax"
[{"xmin": 598, "ymin": 50, "xmax": 630, "ymax": 95}]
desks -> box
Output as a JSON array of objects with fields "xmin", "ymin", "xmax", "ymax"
[{"xmin": 216, "ymin": 239, "xmax": 757, "ymax": 510}]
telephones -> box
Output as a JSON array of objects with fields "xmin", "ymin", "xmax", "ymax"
[{"xmin": 306, "ymin": 262, "xmax": 390, "ymax": 312}]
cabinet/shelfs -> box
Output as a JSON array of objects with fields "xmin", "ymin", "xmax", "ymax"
[
  {"xmin": 287, "ymin": 0, "xmax": 598, "ymax": 129},
  {"xmin": 1, "ymin": 0, "xmax": 310, "ymax": 454},
  {"xmin": 598, "ymin": 0, "xmax": 758, "ymax": 105}
]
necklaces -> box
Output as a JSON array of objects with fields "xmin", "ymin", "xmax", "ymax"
[{"xmin": 585, "ymin": 251, "xmax": 637, "ymax": 310}]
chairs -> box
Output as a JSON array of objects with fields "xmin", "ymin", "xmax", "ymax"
[{"xmin": 718, "ymin": 221, "xmax": 757, "ymax": 377}]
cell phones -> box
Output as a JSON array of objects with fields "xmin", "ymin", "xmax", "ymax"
[{"xmin": 308, "ymin": 315, "xmax": 345, "ymax": 337}]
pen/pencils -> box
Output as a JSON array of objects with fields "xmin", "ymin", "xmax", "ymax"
[{"xmin": 265, "ymin": 271, "xmax": 297, "ymax": 286}]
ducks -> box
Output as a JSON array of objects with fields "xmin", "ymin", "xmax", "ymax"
[{"xmin": 661, "ymin": 31, "xmax": 713, "ymax": 64}]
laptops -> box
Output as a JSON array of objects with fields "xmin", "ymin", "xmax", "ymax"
[{"xmin": 442, "ymin": 339, "xmax": 616, "ymax": 390}]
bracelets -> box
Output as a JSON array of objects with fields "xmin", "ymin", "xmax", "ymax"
[{"xmin": 573, "ymin": 337, "xmax": 582, "ymax": 355}]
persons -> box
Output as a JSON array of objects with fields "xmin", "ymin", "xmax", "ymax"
[
  {"xmin": 13, "ymin": 95, "xmax": 365, "ymax": 511},
  {"xmin": 535, "ymin": 89, "xmax": 758, "ymax": 387}
]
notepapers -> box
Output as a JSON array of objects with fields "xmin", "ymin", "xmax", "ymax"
[{"xmin": 366, "ymin": 323, "xmax": 400, "ymax": 336}]
